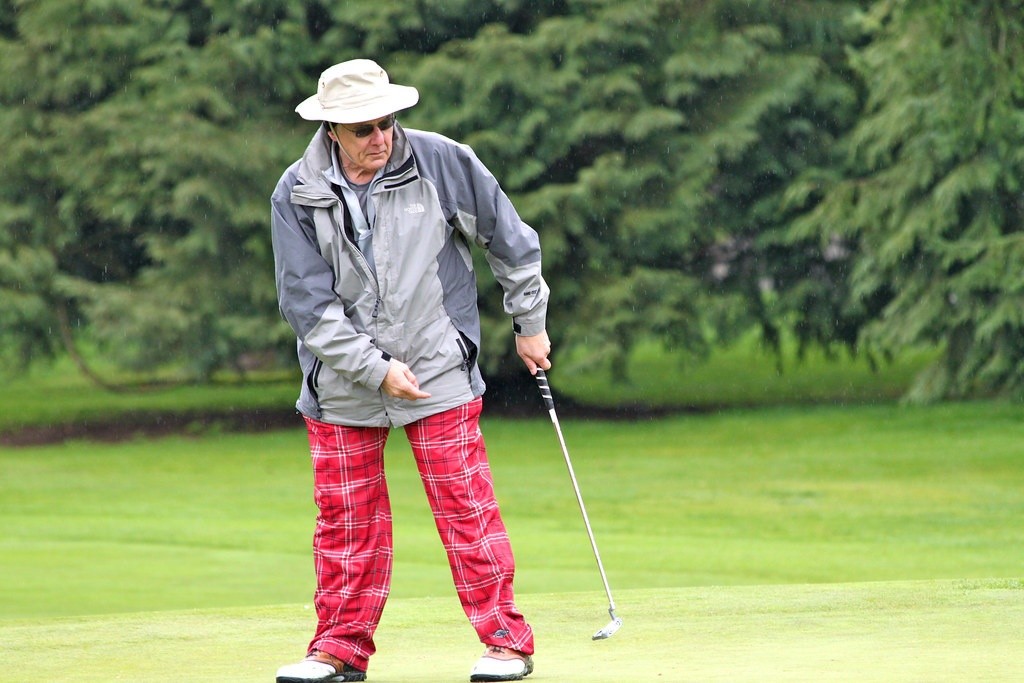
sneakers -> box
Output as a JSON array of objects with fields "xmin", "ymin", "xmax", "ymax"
[
  {"xmin": 275, "ymin": 650, "xmax": 367, "ymax": 683},
  {"xmin": 470, "ymin": 645, "xmax": 534, "ymax": 682}
]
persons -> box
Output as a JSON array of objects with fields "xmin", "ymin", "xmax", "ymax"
[{"xmin": 270, "ymin": 73, "xmax": 554, "ymax": 683}]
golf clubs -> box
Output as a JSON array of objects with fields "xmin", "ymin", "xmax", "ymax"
[{"xmin": 534, "ymin": 362, "xmax": 620, "ymax": 640}]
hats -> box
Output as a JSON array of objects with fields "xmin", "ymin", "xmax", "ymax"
[{"xmin": 294, "ymin": 58, "xmax": 419, "ymax": 124}]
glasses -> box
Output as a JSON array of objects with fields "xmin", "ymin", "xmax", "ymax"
[{"xmin": 340, "ymin": 115, "xmax": 397, "ymax": 138}]
